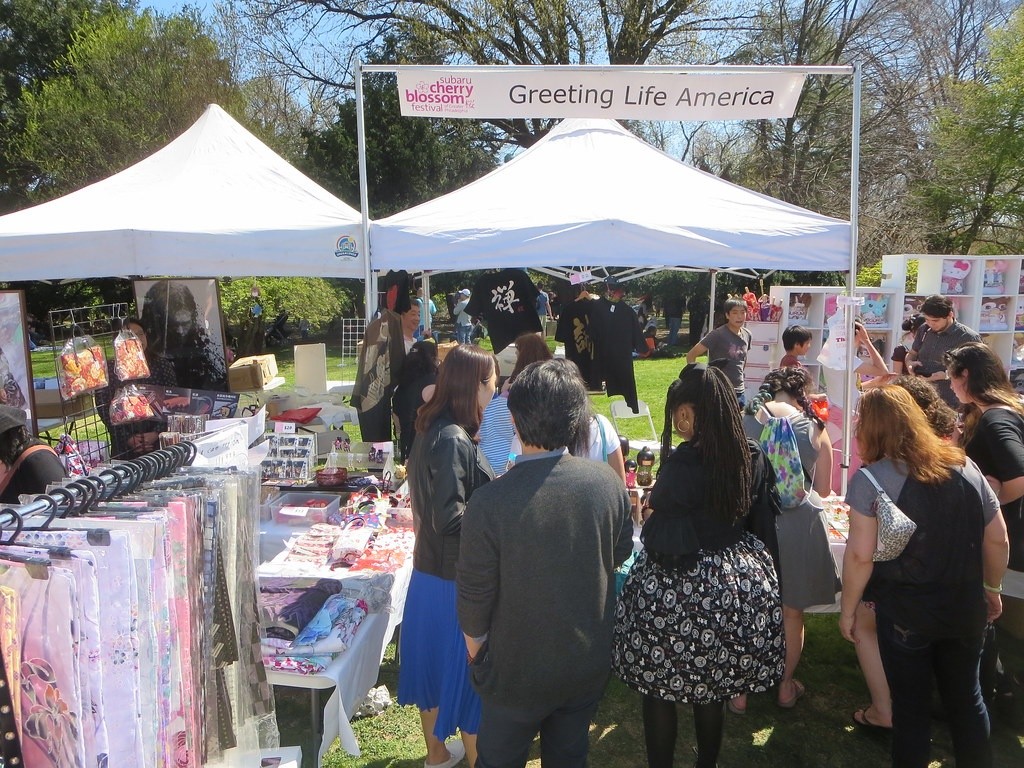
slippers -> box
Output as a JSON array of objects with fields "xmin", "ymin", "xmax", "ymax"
[
  {"xmin": 853, "ymin": 706, "xmax": 896, "ymax": 733},
  {"xmin": 777, "ymin": 679, "xmax": 804, "ymax": 707},
  {"xmin": 727, "ymin": 700, "xmax": 745, "ymax": 714}
]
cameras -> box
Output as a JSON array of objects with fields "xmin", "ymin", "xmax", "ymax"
[{"xmin": 914, "ymin": 368, "xmax": 932, "ymax": 377}]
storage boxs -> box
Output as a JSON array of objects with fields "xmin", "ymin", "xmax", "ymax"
[
  {"xmin": 268, "ymin": 493, "xmax": 341, "ymax": 523},
  {"xmin": 228, "ymin": 353, "xmax": 278, "ymax": 390},
  {"xmin": 34, "ymin": 388, "xmax": 94, "ymax": 418},
  {"xmin": 260, "ymin": 487, "xmax": 280, "ymax": 522}
]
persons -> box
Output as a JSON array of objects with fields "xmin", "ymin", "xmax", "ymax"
[
  {"xmin": 838, "ymin": 387, "xmax": 1010, "ymax": 768},
  {"xmin": 475, "ymin": 332, "xmax": 625, "ymax": 483},
  {"xmin": 459, "ymin": 359, "xmax": 633, "ymax": 768},
  {"xmin": 943, "ymin": 341, "xmax": 1024, "ymax": 734},
  {"xmin": 781, "ymin": 324, "xmax": 813, "ymax": 370},
  {"xmin": 93, "ymin": 317, "xmax": 178, "ymax": 461},
  {"xmin": 612, "ymin": 363, "xmax": 786, "ymax": 768},
  {"xmin": 141, "ymin": 280, "xmax": 229, "ymax": 394},
  {"xmin": 727, "ymin": 363, "xmax": 842, "ymax": 713},
  {"xmin": 299, "ymin": 315, "xmax": 311, "ymax": 343},
  {"xmin": 224, "ymin": 331, "xmax": 235, "ymax": 370},
  {"xmin": 687, "ymin": 294, "xmax": 751, "ymax": 412},
  {"xmin": 0, "ymin": 403, "xmax": 72, "ymax": 504},
  {"xmin": 822, "ymin": 318, "xmax": 890, "ymax": 495},
  {"xmin": 959, "ymin": 401, "xmax": 984, "ymax": 449},
  {"xmin": 891, "ymin": 313, "xmax": 927, "ymax": 376},
  {"xmin": 409, "ymin": 267, "xmax": 709, "ymax": 358},
  {"xmin": 395, "ymin": 344, "xmax": 499, "ymax": 768},
  {"xmin": 905, "ymin": 294, "xmax": 984, "ymax": 409},
  {"xmin": 349, "ymin": 281, "xmax": 442, "ymax": 466},
  {"xmin": 894, "ymin": 372, "xmax": 959, "ymax": 440}
]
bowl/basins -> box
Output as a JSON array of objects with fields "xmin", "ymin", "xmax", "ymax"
[{"xmin": 316, "ymin": 468, "xmax": 347, "ymax": 486}]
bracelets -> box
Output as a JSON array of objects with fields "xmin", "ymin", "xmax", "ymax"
[
  {"xmin": 945, "ymin": 369, "xmax": 949, "ymax": 380},
  {"xmin": 984, "ymin": 581, "xmax": 1002, "ymax": 594},
  {"xmin": 909, "ymin": 365, "xmax": 913, "ymax": 369}
]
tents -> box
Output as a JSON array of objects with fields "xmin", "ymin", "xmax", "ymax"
[
  {"xmin": 0, "ymin": 104, "xmax": 371, "ymax": 324},
  {"xmin": 370, "ymin": 118, "xmax": 858, "ymax": 503}
]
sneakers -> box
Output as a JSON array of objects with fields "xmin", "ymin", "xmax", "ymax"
[{"xmin": 424, "ymin": 739, "xmax": 466, "ymax": 768}]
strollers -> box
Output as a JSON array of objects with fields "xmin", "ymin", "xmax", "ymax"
[{"xmin": 265, "ymin": 309, "xmax": 293, "ymax": 347}]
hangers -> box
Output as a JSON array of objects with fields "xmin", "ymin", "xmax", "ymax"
[
  {"xmin": 0, "ymin": 441, "xmax": 237, "ymax": 580},
  {"xmin": 575, "ymin": 277, "xmax": 620, "ymax": 300}
]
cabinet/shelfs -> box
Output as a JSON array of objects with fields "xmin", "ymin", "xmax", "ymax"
[{"xmin": 770, "ymin": 252, "xmax": 1024, "ymax": 387}]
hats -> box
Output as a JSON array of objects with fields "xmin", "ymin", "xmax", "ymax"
[
  {"xmin": 0, "ymin": 404, "xmax": 27, "ymax": 434},
  {"xmin": 459, "ymin": 289, "xmax": 471, "ymax": 296}
]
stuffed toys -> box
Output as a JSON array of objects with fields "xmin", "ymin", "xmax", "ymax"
[
  {"xmin": 980, "ymin": 295, "xmax": 1024, "ymax": 394},
  {"xmin": 940, "ymin": 259, "xmax": 1024, "ymax": 296},
  {"xmin": 789, "ymin": 292, "xmax": 890, "ymax": 328}
]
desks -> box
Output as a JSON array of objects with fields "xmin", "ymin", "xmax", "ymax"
[
  {"xmin": 36, "ymin": 409, "xmax": 98, "ymax": 454},
  {"xmin": 259, "ymin": 522, "xmax": 415, "ymax": 768},
  {"xmin": 803, "ymin": 507, "xmax": 849, "ymax": 615},
  {"xmin": 233, "ymin": 376, "xmax": 285, "ymax": 394}
]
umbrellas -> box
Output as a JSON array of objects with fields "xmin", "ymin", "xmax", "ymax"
[{"xmin": 726, "ymin": 284, "xmax": 784, "ymax": 322}]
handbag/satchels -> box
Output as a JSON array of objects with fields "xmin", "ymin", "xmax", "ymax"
[
  {"xmin": 54, "ymin": 422, "xmax": 90, "ymax": 478},
  {"xmin": 860, "ymin": 467, "xmax": 917, "ymax": 562},
  {"xmin": 110, "ymin": 385, "xmax": 154, "ymax": 427},
  {"xmin": 113, "ymin": 316, "xmax": 151, "ymax": 381},
  {"xmin": 57, "ymin": 325, "xmax": 109, "ymax": 402}
]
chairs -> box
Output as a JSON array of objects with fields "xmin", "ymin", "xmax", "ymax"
[{"xmin": 609, "ymin": 400, "xmax": 678, "ymax": 466}]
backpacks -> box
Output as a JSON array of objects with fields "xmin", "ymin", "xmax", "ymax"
[{"xmin": 760, "ymin": 403, "xmax": 811, "ymax": 510}]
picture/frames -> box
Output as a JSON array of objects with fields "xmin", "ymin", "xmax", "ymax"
[{"xmin": 0, "ymin": 290, "xmax": 38, "ymax": 439}]
